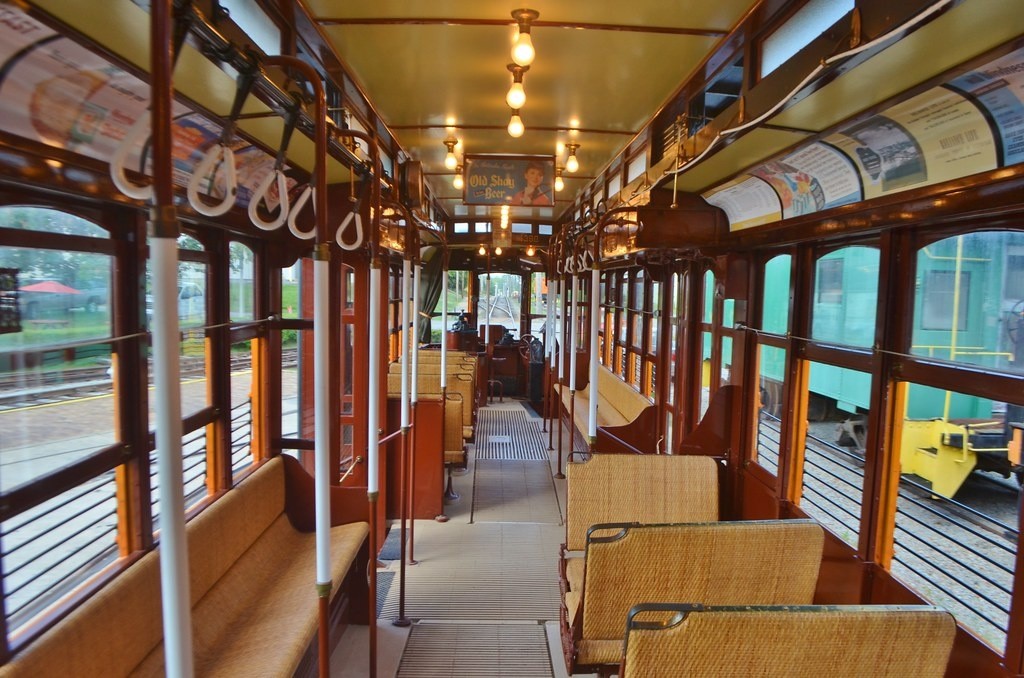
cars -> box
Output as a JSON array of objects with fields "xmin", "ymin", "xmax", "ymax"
[
  {"xmin": 601, "ymin": 327, "xmax": 677, "ymax": 380},
  {"xmin": 15, "ymin": 276, "xmax": 109, "ymax": 317},
  {"xmin": 145, "ymin": 283, "xmax": 208, "ymax": 320}
]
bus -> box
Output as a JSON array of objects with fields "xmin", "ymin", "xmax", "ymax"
[{"xmin": 703, "ymin": 231, "xmax": 1024, "ymax": 500}]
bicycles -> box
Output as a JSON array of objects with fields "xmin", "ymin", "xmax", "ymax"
[{"xmin": 534, "ymin": 276, "xmax": 558, "ymax": 305}]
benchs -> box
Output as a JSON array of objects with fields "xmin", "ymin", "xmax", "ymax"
[
  {"xmin": 1, "ymin": 349, "xmax": 479, "ymax": 678},
  {"xmin": 553, "ymin": 364, "xmax": 956, "ymax": 678}
]
